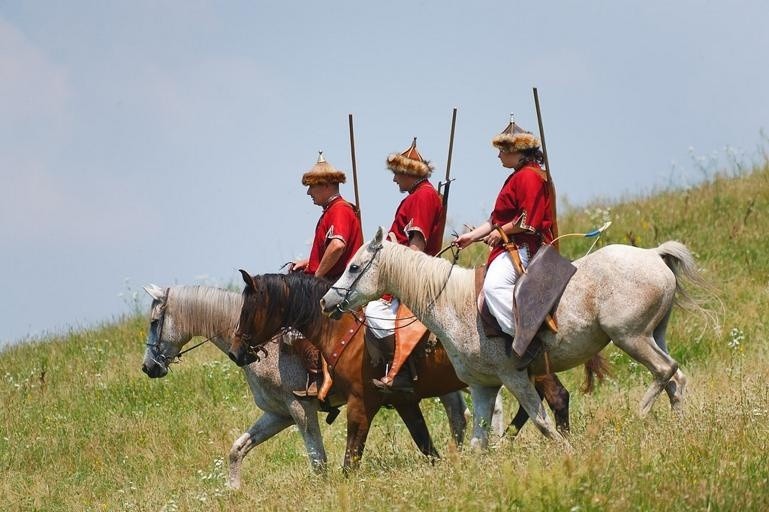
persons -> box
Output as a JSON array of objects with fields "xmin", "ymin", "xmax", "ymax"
[
  {"xmin": 288, "ymin": 148, "xmax": 360, "ymax": 400},
  {"xmin": 453, "ymin": 112, "xmax": 556, "ymax": 371},
  {"xmin": 364, "ymin": 136, "xmax": 441, "ymax": 395}
]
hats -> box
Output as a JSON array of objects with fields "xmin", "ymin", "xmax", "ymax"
[
  {"xmin": 387, "ymin": 137, "xmax": 433, "ymax": 178},
  {"xmin": 302, "ymin": 149, "xmax": 345, "ymax": 186},
  {"xmin": 492, "ymin": 112, "xmax": 542, "ymax": 154}
]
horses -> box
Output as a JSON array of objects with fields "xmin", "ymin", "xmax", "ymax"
[
  {"xmin": 140, "ymin": 283, "xmax": 505, "ymax": 490},
  {"xmin": 231, "ymin": 269, "xmax": 625, "ymax": 483},
  {"xmin": 319, "ymin": 225, "xmax": 726, "ymax": 456}
]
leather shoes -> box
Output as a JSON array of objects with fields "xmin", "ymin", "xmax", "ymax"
[
  {"xmin": 370, "ymin": 375, "xmax": 414, "ymax": 394},
  {"xmin": 292, "ymin": 379, "xmax": 336, "ymax": 399},
  {"xmin": 516, "ymin": 338, "xmax": 546, "ymax": 372}
]
